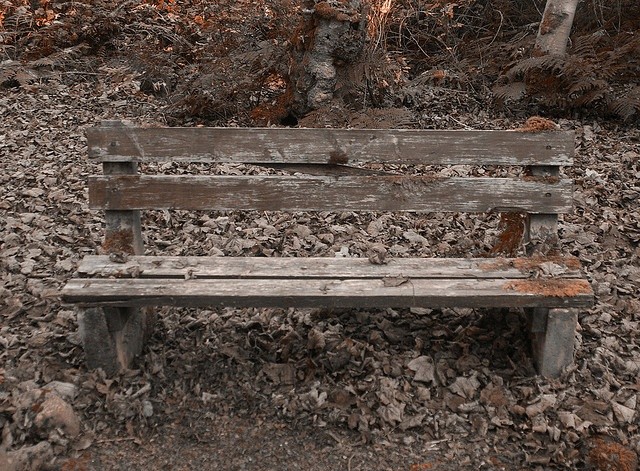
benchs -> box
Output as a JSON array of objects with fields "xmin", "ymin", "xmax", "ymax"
[{"xmin": 59, "ymin": 120, "xmax": 595, "ymax": 379}]
